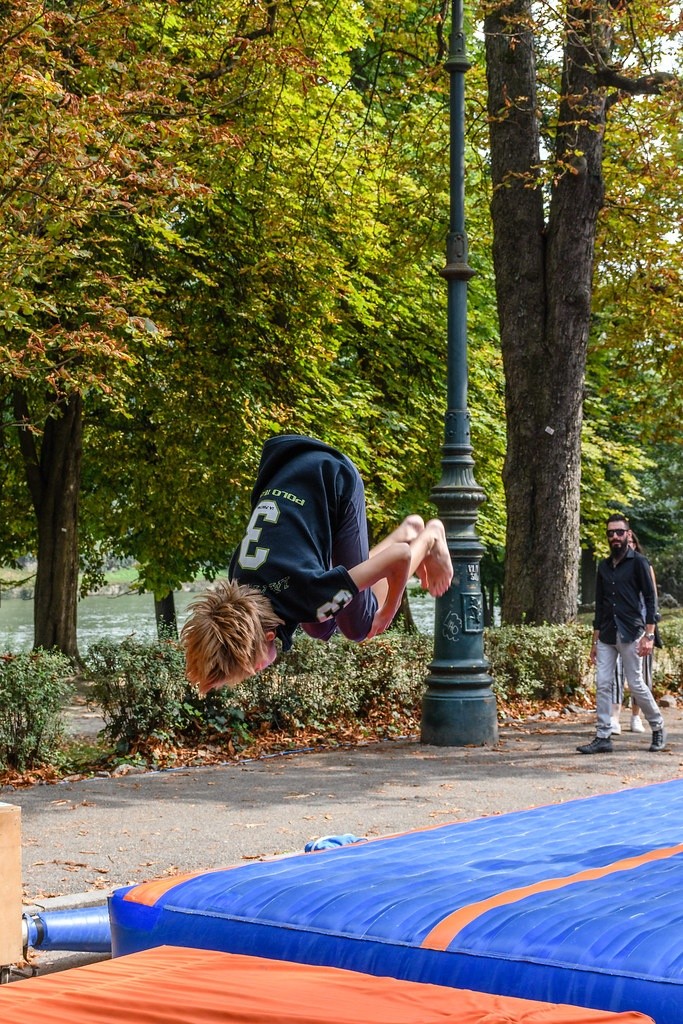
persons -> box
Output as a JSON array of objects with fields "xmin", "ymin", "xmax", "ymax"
[
  {"xmin": 608, "ymin": 530, "xmax": 665, "ymax": 737},
  {"xmin": 577, "ymin": 513, "xmax": 667, "ymax": 756},
  {"xmin": 179, "ymin": 429, "xmax": 454, "ymax": 698}
]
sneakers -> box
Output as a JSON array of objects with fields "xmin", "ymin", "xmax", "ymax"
[
  {"xmin": 594, "ymin": 718, "xmax": 622, "ymax": 735},
  {"xmin": 630, "ymin": 715, "xmax": 644, "ymax": 733},
  {"xmin": 576, "ymin": 736, "xmax": 613, "ymax": 753},
  {"xmin": 650, "ymin": 726, "xmax": 668, "ymax": 752}
]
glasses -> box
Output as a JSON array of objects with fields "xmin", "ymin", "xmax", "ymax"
[
  {"xmin": 628, "ymin": 540, "xmax": 635, "ymax": 543},
  {"xmin": 606, "ymin": 528, "xmax": 629, "ymax": 537}
]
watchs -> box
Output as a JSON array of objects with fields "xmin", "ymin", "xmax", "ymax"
[{"xmin": 643, "ymin": 633, "xmax": 655, "ymax": 641}]
